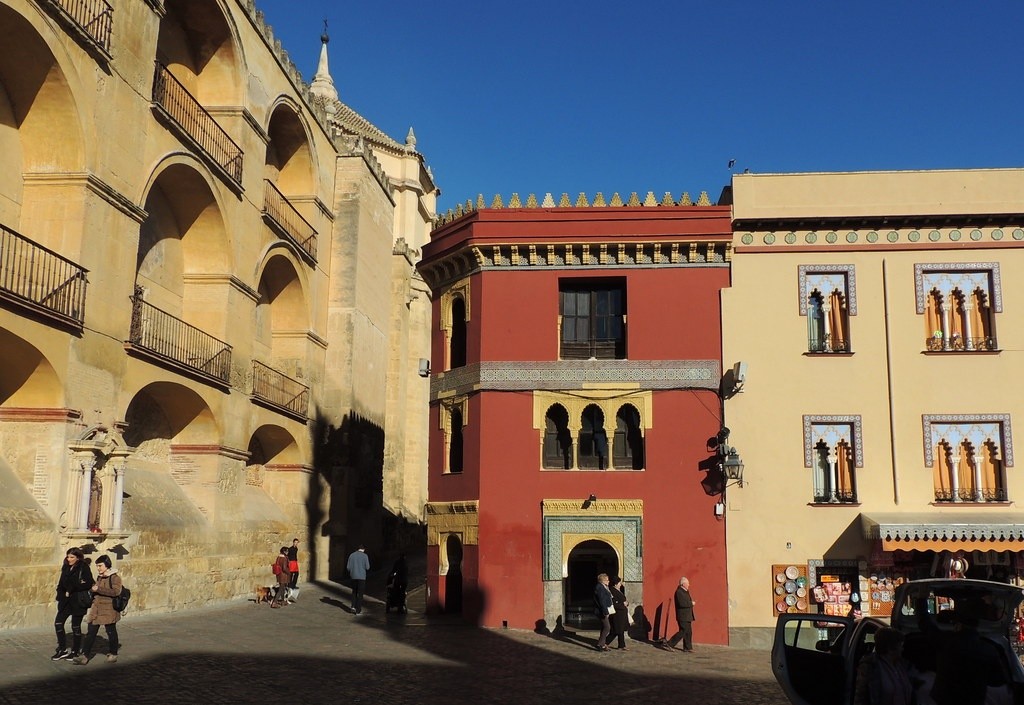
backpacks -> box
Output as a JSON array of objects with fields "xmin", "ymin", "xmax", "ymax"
[{"xmin": 271, "ymin": 564, "xmax": 282, "ymax": 574}]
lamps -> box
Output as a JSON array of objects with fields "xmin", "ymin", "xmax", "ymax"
[
  {"xmin": 589, "ymin": 494, "xmax": 596, "ymax": 503},
  {"xmin": 733, "ymin": 361, "xmax": 746, "ymax": 392},
  {"xmin": 724, "ymin": 447, "xmax": 743, "ymax": 488},
  {"xmin": 418, "ymin": 358, "xmax": 431, "ymax": 376}
]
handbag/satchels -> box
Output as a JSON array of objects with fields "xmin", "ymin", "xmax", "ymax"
[
  {"xmin": 607, "ymin": 605, "xmax": 616, "ymax": 615},
  {"xmin": 77, "ymin": 563, "xmax": 94, "ymax": 608},
  {"xmin": 289, "ymin": 561, "xmax": 299, "ymax": 573},
  {"xmin": 627, "ymin": 607, "xmax": 635, "ymax": 624},
  {"xmin": 109, "ymin": 573, "xmax": 131, "ymax": 612}
]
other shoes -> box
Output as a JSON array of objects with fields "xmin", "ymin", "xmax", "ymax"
[
  {"xmin": 271, "ymin": 603, "xmax": 282, "ymax": 608},
  {"xmin": 617, "ymin": 646, "xmax": 631, "ymax": 652},
  {"xmin": 73, "ymin": 654, "xmax": 89, "ymax": 665},
  {"xmin": 352, "ymin": 607, "xmax": 363, "ymax": 615},
  {"xmin": 666, "ymin": 642, "xmax": 675, "ymax": 652},
  {"xmin": 603, "ymin": 643, "xmax": 611, "ymax": 651},
  {"xmin": 684, "ymin": 648, "xmax": 695, "ymax": 653},
  {"xmin": 105, "ymin": 653, "xmax": 118, "ymax": 663},
  {"xmin": 597, "ymin": 645, "xmax": 604, "ymax": 651},
  {"xmin": 281, "ymin": 600, "xmax": 288, "ymax": 607}
]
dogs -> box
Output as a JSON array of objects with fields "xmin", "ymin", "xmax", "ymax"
[
  {"xmin": 255, "ymin": 586, "xmax": 270, "ymax": 603},
  {"xmin": 272, "ymin": 582, "xmax": 295, "ymax": 604}
]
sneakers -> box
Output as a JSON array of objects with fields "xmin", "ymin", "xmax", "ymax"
[
  {"xmin": 65, "ymin": 651, "xmax": 80, "ymax": 661},
  {"xmin": 52, "ymin": 647, "xmax": 69, "ymax": 660}
]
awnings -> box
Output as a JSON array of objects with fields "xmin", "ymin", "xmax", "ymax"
[{"xmin": 861, "ymin": 512, "xmax": 1024, "ymax": 552}]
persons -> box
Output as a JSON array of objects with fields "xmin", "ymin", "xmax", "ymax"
[
  {"xmin": 347, "ymin": 542, "xmax": 370, "ymax": 615},
  {"xmin": 386, "ymin": 572, "xmax": 392, "ymax": 594},
  {"xmin": 392, "ymin": 552, "xmax": 408, "ymax": 615},
  {"xmin": 288, "ymin": 538, "xmax": 299, "ymax": 589},
  {"xmin": 855, "ymin": 626, "xmax": 913, "ymax": 705},
  {"xmin": 605, "ymin": 576, "xmax": 629, "ymax": 651},
  {"xmin": 593, "ymin": 572, "xmax": 616, "ymax": 652},
  {"xmin": 72, "ymin": 555, "xmax": 123, "ymax": 664},
  {"xmin": 51, "ymin": 547, "xmax": 93, "ymax": 662},
  {"xmin": 270, "ymin": 547, "xmax": 292, "ymax": 607},
  {"xmin": 662, "ymin": 577, "xmax": 696, "ymax": 653}
]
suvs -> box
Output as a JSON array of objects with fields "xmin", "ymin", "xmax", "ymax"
[{"xmin": 771, "ymin": 579, "xmax": 1024, "ymax": 705}]
